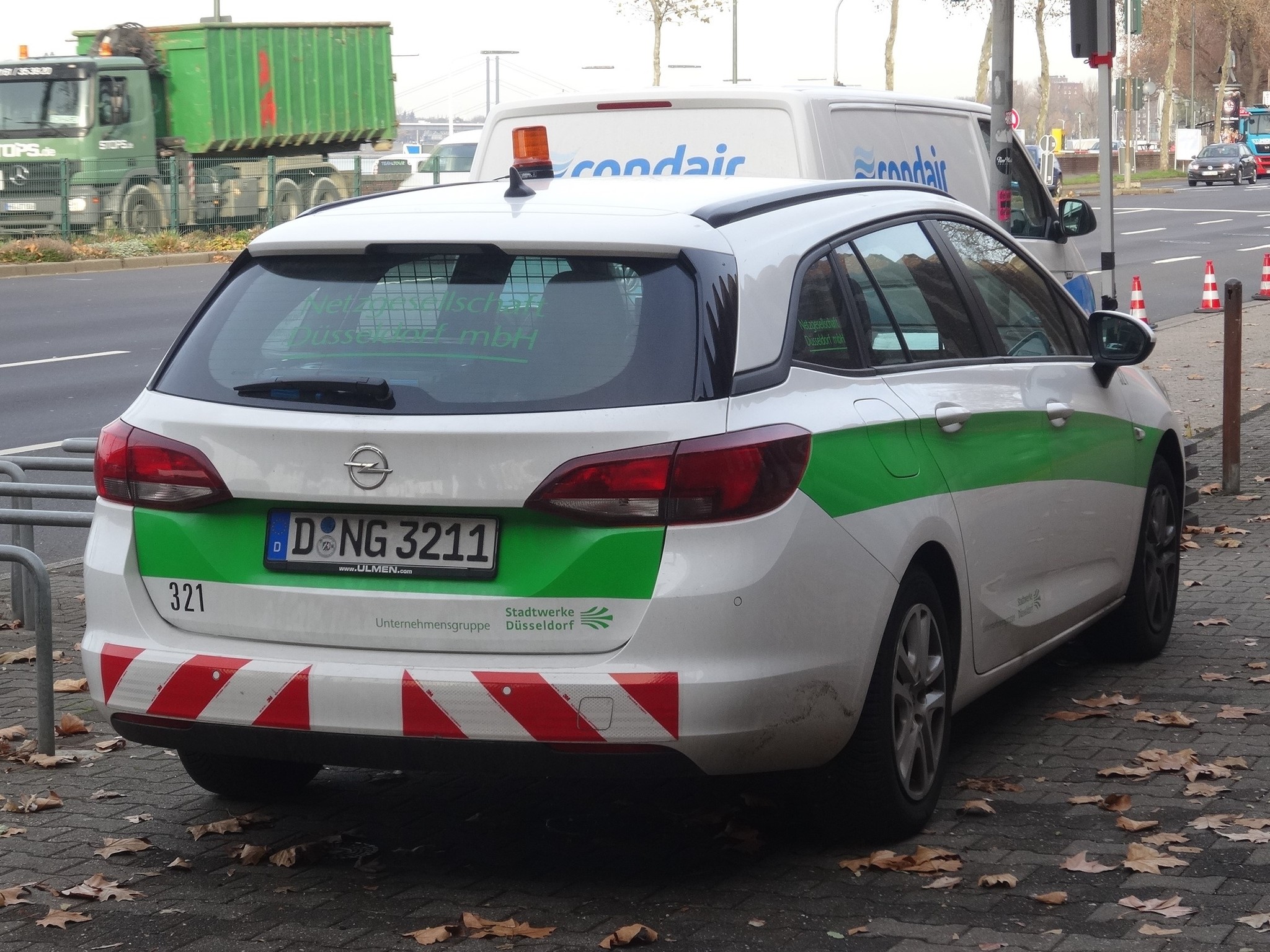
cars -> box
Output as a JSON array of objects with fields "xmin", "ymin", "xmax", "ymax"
[
  {"xmin": 398, "ymin": 129, "xmax": 483, "ymax": 190},
  {"xmin": 1187, "ymin": 141, "xmax": 1257, "ymax": 187},
  {"xmin": 80, "ymin": 123, "xmax": 1190, "ymax": 839},
  {"xmin": 1011, "ymin": 145, "xmax": 1063, "ymax": 198},
  {"xmin": 1085, "ymin": 139, "xmax": 1126, "ymax": 154}
]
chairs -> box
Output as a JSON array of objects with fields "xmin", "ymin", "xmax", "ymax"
[
  {"xmin": 798, "ymin": 275, "xmax": 884, "ymax": 367},
  {"xmin": 520, "ymin": 272, "xmax": 640, "ymax": 400}
]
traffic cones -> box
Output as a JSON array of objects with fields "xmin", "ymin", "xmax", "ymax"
[
  {"xmin": 1127, "ymin": 275, "xmax": 1158, "ymax": 330},
  {"xmin": 1193, "ymin": 260, "xmax": 1227, "ymax": 313},
  {"xmin": 1251, "ymin": 253, "xmax": 1269, "ymax": 300}
]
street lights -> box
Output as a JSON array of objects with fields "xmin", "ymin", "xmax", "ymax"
[{"xmin": 1143, "ymin": 77, "xmax": 1156, "ymax": 148}]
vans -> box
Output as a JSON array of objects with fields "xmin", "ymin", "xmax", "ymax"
[
  {"xmin": 372, "ymin": 153, "xmax": 450, "ymax": 173},
  {"xmin": 467, "ymin": 83, "xmax": 1110, "ymax": 329}
]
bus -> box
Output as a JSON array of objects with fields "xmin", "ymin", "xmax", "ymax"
[{"xmin": 1234, "ymin": 104, "xmax": 1270, "ymax": 180}]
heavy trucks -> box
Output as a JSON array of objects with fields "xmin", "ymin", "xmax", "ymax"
[{"xmin": 0, "ymin": 20, "xmax": 402, "ymax": 236}]
